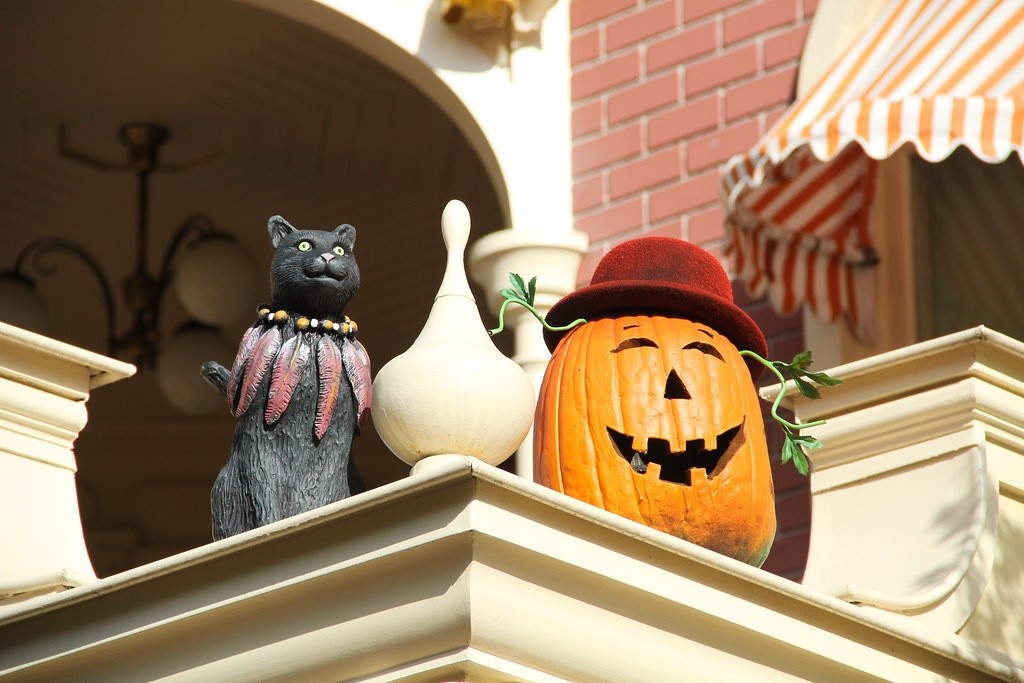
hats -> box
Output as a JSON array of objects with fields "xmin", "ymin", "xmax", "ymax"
[{"xmin": 543, "ymin": 237, "xmax": 766, "ymax": 385}]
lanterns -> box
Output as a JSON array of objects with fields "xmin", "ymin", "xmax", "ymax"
[{"xmin": 534, "ymin": 312, "xmax": 778, "ymax": 569}]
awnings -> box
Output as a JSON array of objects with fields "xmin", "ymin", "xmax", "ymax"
[{"xmin": 720, "ymin": 0, "xmax": 1024, "ymax": 343}]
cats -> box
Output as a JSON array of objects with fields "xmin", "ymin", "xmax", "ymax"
[{"xmin": 200, "ymin": 216, "xmax": 372, "ymax": 544}]
